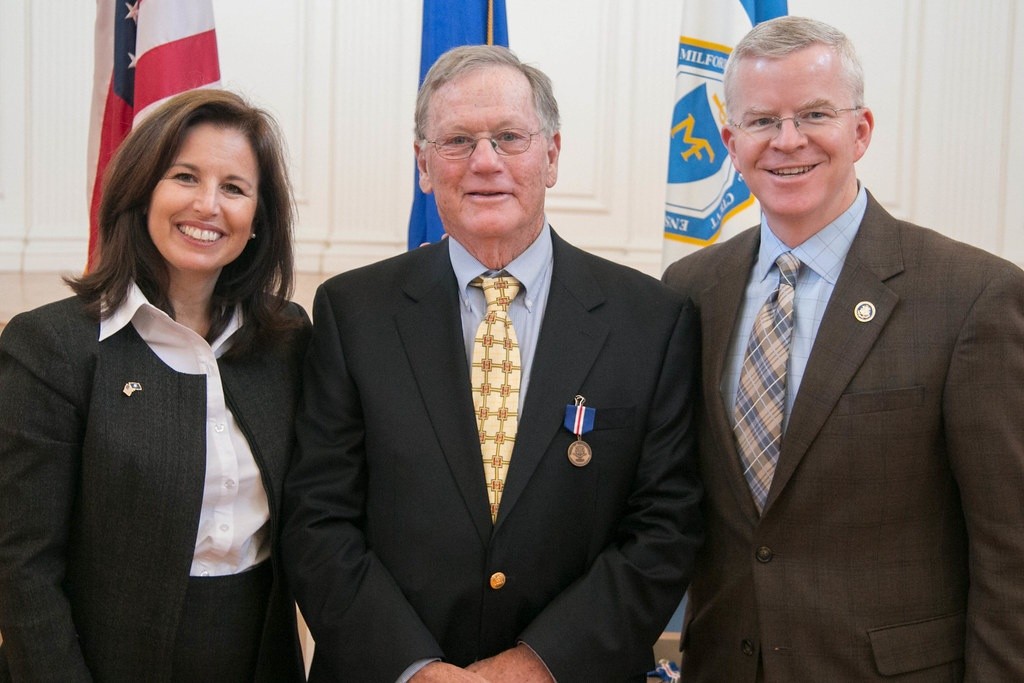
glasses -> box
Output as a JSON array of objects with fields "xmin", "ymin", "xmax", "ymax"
[
  {"xmin": 421, "ymin": 128, "xmax": 544, "ymax": 160},
  {"xmin": 724, "ymin": 105, "xmax": 866, "ymax": 141}
]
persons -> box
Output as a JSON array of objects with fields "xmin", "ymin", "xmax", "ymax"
[
  {"xmin": 280, "ymin": 44, "xmax": 704, "ymax": 682},
  {"xmin": 417, "ymin": 16, "xmax": 1024, "ymax": 683},
  {"xmin": 0, "ymin": 90, "xmax": 313, "ymax": 683}
]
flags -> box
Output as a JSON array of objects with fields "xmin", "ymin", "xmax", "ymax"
[
  {"xmin": 81, "ymin": 1, "xmax": 222, "ymax": 278},
  {"xmin": 407, "ymin": 0, "xmax": 509, "ymax": 251},
  {"xmin": 660, "ymin": 0, "xmax": 787, "ymax": 280}
]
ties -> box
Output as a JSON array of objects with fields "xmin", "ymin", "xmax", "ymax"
[
  {"xmin": 732, "ymin": 251, "xmax": 803, "ymax": 516},
  {"xmin": 469, "ymin": 270, "xmax": 523, "ymax": 520}
]
